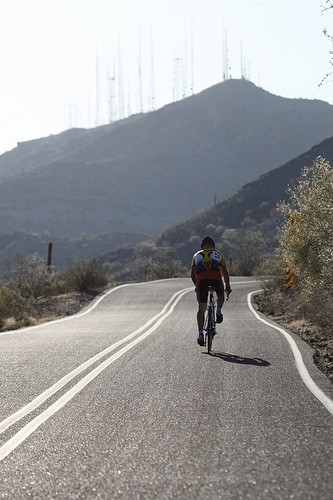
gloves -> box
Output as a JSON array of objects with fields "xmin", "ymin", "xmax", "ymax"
[{"xmin": 226, "ymin": 287, "xmax": 231, "ymax": 294}]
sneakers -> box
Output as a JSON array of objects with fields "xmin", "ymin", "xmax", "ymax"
[
  {"xmin": 216, "ymin": 311, "xmax": 223, "ymax": 323},
  {"xmin": 199, "ymin": 333, "xmax": 204, "ymax": 346}
]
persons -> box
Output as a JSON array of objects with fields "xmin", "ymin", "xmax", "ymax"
[{"xmin": 190, "ymin": 237, "xmax": 231, "ymax": 345}]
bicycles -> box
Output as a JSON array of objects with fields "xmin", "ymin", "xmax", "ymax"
[{"xmin": 200, "ymin": 286, "xmax": 230, "ymax": 354}]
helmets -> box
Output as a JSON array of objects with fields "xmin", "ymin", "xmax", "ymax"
[{"xmin": 201, "ymin": 237, "xmax": 215, "ymax": 249}]
